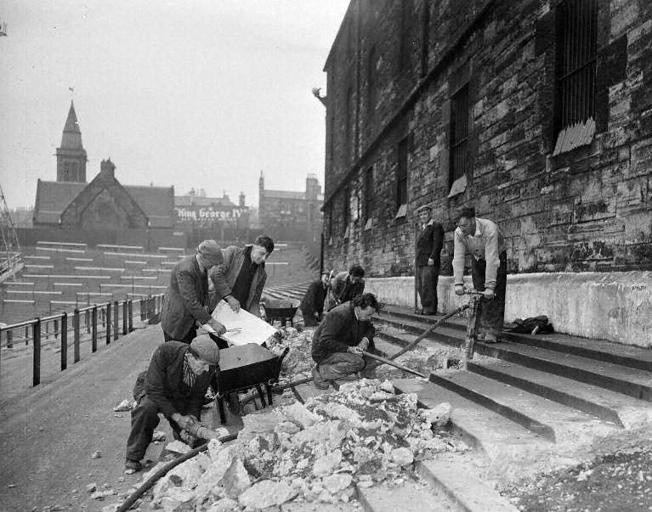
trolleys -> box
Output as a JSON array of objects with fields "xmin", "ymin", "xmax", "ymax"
[
  {"xmin": 205, "ymin": 339, "xmax": 292, "ymax": 425},
  {"xmin": 261, "ymin": 296, "xmax": 301, "ymax": 325}
]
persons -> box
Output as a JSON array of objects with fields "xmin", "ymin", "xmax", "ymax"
[
  {"xmin": 161, "ymin": 239, "xmax": 227, "ymax": 344},
  {"xmin": 300, "ymin": 269, "xmax": 330, "ymax": 326},
  {"xmin": 208, "ymin": 236, "xmax": 275, "ymax": 315},
  {"xmin": 124, "ymin": 334, "xmax": 220, "ymax": 472},
  {"xmin": 311, "ymin": 292, "xmax": 379, "ymax": 389},
  {"xmin": 323, "ymin": 267, "xmax": 364, "ymax": 314},
  {"xmin": 454, "ymin": 207, "xmax": 507, "ymax": 344},
  {"xmin": 415, "ymin": 206, "xmax": 444, "ymax": 316}
]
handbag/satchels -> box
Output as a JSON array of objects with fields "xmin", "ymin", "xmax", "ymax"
[{"xmin": 508, "ymin": 315, "xmax": 550, "ymax": 335}]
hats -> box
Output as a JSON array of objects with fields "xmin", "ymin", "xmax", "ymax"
[
  {"xmin": 198, "ymin": 239, "xmax": 223, "ymax": 266},
  {"xmin": 192, "ymin": 337, "xmax": 219, "ymax": 366},
  {"xmin": 418, "ymin": 205, "xmax": 432, "ymax": 212}
]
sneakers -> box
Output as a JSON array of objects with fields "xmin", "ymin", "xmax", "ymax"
[
  {"xmin": 125, "ymin": 458, "xmax": 140, "ymax": 469},
  {"xmin": 477, "ymin": 333, "xmax": 501, "ymax": 343},
  {"xmin": 311, "ymin": 368, "xmax": 329, "ymax": 389}
]
